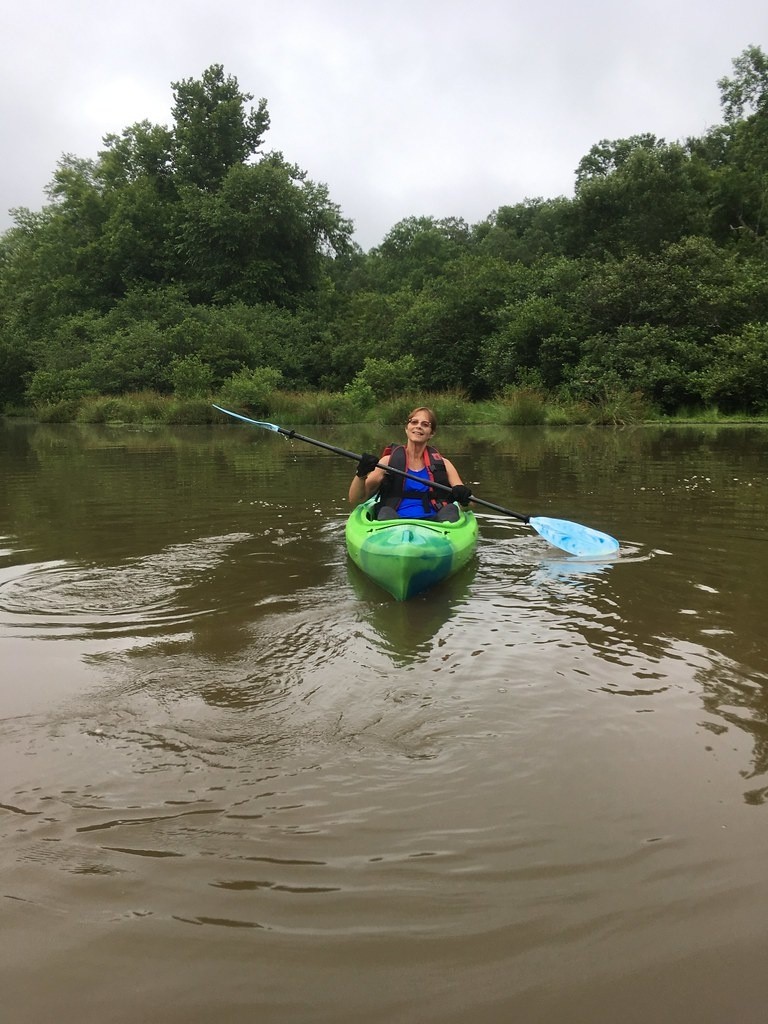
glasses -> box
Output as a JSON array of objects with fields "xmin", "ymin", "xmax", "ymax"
[{"xmin": 408, "ymin": 418, "xmax": 430, "ymax": 428}]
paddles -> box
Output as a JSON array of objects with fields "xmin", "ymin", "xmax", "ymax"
[{"xmin": 210, "ymin": 400, "xmax": 620, "ymax": 558}]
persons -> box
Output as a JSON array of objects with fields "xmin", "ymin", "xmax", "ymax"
[{"xmin": 349, "ymin": 407, "xmax": 475, "ymax": 523}]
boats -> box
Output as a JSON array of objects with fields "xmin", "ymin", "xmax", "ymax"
[{"xmin": 345, "ymin": 494, "xmax": 478, "ymax": 602}]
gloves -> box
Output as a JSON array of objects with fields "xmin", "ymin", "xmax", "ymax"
[
  {"xmin": 447, "ymin": 485, "xmax": 472, "ymax": 507},
  {"xmin": 355, "ymin": 453, "xmax": 379, "ymax": 478}
]
